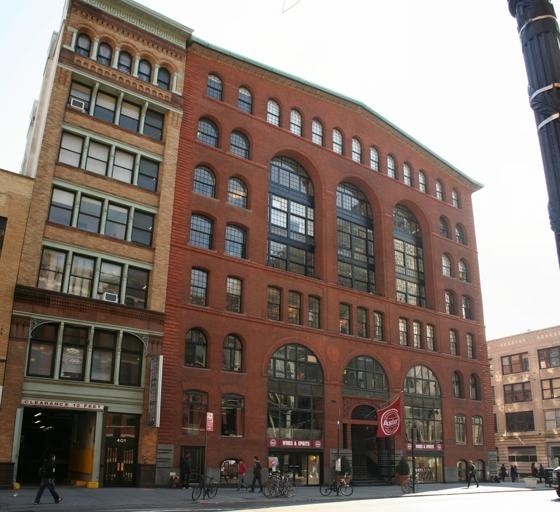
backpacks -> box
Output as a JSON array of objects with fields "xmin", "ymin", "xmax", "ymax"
[{"xmin": 39, "ymin": 463, "xmax": 51, "ymax": 478}]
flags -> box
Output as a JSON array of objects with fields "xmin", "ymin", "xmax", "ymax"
[{"xmin": 376, "ymin": 393, "xmax": 402, "ymax": 438}]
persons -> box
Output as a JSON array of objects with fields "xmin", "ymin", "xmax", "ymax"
[
  {"xmin": 467, "ymin": 459, "xmax": 479, "ymax": 488},
  {"xmin": 531, "ymin": 463, "xmax": 544, "ymax": 482},
  {"xmin": 182, "ymin": 450, "xmax": 192, "ymax": 490},
  {"xmin": 236, "ymin": 459, "xmax": 248, "ymax": 492},
  {"xmin": 33, "ymin": 452, "xmax": 65, "ymax": 505},
  {"xmin": 396, "ymin": 456, "xmax": 410, "ymax": 490},
  {"xmin": 249, "ymin": 456, "xmax": 263, "ymax": 493},
  {"xmin": 500, "ymin": 463, "xmax": 518, "ymax": 482}
]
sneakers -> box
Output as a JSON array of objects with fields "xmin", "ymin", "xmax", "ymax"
[{"xmin": 55, "ymin": 497, "xmax": 63, "ymax": 504}]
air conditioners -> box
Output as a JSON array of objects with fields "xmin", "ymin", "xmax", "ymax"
[{"xmin": 68, "ymin": 98, "xmax": 86, "ymax": 113}]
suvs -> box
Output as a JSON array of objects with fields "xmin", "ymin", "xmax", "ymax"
[{"xmin": 544, "ymin": 467, "xmax": 560, "ymax": 497}]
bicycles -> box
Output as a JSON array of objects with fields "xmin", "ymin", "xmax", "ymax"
[
  {"xmin": 262, "ymin": 473, "xmax": 297, "ymax": 500},
  {"xmin": 319, "ymin": 476, "xmax": 353, "ymax": 498},
  {"xmin": 192, "ymin": 474, "xmax": 218, "ymax": 501},
  {"xmin": 400, "ymin": 475, "xmax": 419, "ymax": 493}
]
